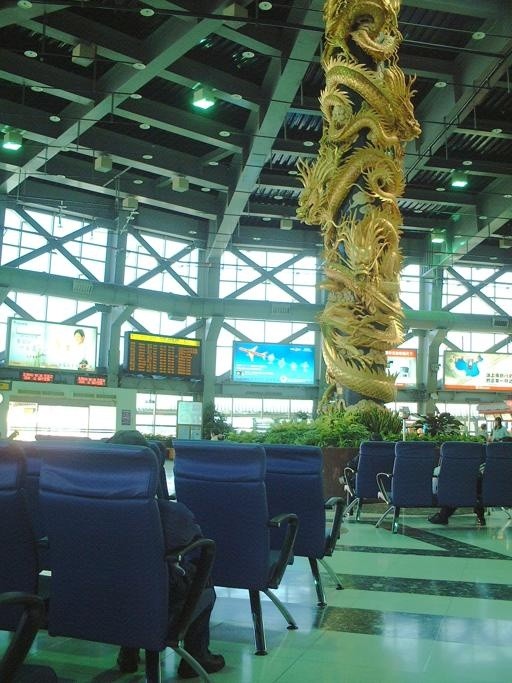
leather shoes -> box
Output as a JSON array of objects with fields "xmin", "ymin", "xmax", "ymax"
[
  {"xmin": 428, "ymin": 513, "xmax": 448, "ymax": 524},
  {"xmin": 117, "ymin": 651, "xmax": 140, "ymax": 671},
  {"xmin": 476, "ymin": 518, "xmax": 485, "ymax": 525},
  {"xmin": 178, "ymin": 651, "xmax": 224, "ymax": 678}
]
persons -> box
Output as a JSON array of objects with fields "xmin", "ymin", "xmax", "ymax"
[
  {"xmin": 428, "ymin": 465, "xmax": 486, "ymax": 527},
  {"xmin": 479, "ymin": 423, "xmax": 490, "ymax": 441},
  {"xmin": 103, "ymin": 429, "xmax": 225, "ymax": 679},
  {"xmin": 490, "ymin": 416, "xmax": 509, "ymax": 441},
  {"xmin": 68, "ymin": 329, "xmax": 89, "ymax": 365},
  {"xmin": 209, "ymin": 428, "xmax": 223, "ymax": 441},
  {"xmin": 347, "ymin": 431, "xmax": 383, "ymax": 489}
]
